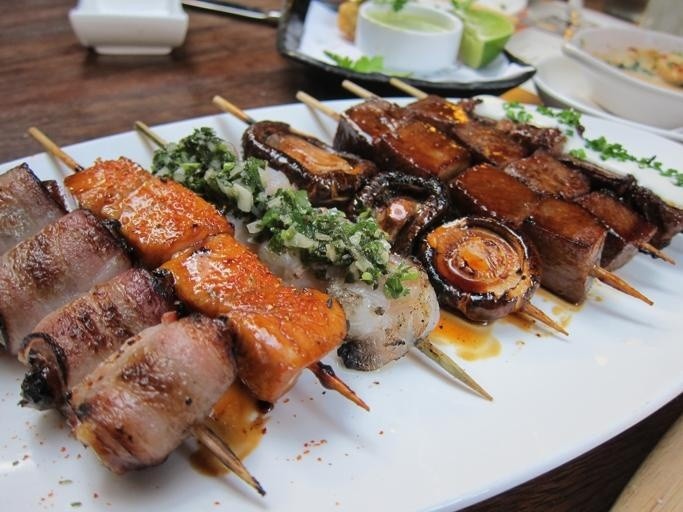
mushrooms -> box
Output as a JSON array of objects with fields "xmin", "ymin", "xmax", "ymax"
[{"xmin": 241, "ymin": 119, "xmax": 544, "ymax": 323}]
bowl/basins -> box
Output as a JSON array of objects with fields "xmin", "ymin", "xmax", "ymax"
[{"xmin": 575, "ymin": 25, "xmax": 683, "ymax": 127}]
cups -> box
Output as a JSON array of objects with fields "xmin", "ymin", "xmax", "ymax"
[{"xmin": 354, "ymin": 2, "xmax": 465, "ymax": 72}]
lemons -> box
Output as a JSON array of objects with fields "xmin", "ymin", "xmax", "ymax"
[{"xmin": 449, "ymin": 7, "xmax": 513, "ymax": 69}]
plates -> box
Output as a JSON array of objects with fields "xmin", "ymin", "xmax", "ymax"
[
  {"xmin": 0, "ymin": 98, "xmax": 683, "ymax": 511},
  {"xmin": 416, "ymin": 1, "xmax": 528, "ymax": 18},
  {"xmin": 66, "ymin": 0, "xmax": 189, "ymax": 56},
  {"xmin": 531, "ymin": 36, "xmax": 682, "ymax": 144}
]
